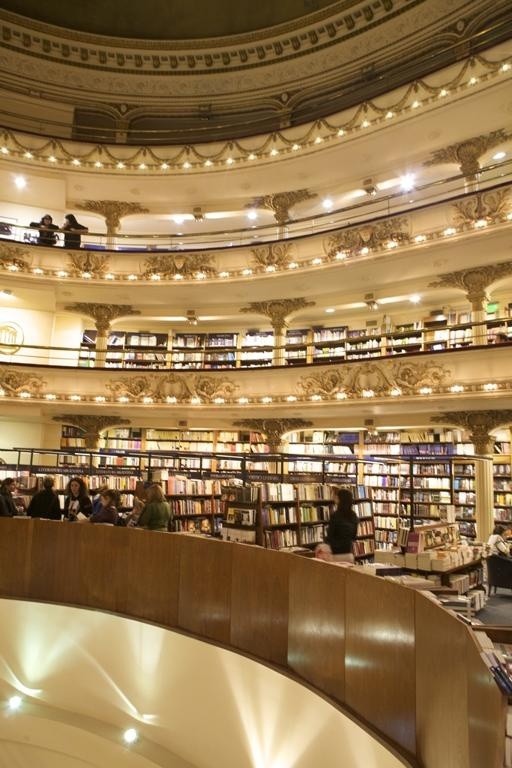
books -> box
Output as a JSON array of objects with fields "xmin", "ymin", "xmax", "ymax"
[
  {"xmin": 347, "ymin": 320, "xmax": 422, "ymax": 359},
  {"xmin": 332, "ymin": 562, "xmax": 510, "ymax": 693},
  {"xmin": 146, "ymin": 430, "xmax": 222, "ymax": 535},
  {"xmin": 28, "ymin": 430, "xmax": 142, "ymax": 517},
  {"xmin": 433, "ymin": 311, "xmax": 510, "ymax": 350},
  {"xmin": 240, "ymin": 330, "xmax": 344, "ymax": 368},
  {"xmin": 364, "ymin": 430, "xmax": 511, "ymax": 572},
  {"xmin": 124, "ymin": 335, "xmax": 237, "ymax": 368},
  {"xmin": 355, "ymin": 485, "xmax": 364, "ymax": 565},
  {"xmin": 79, "ymin": 336, "xmax": 122, "ymax": 369}
]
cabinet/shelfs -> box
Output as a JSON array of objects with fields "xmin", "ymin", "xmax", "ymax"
[
  {"xmin": 0, "ymin": 447, "xmax": 494, "ymax": 616},
  {"xmin": 79, "ymin": 307, "xmax": 512, "ymax": 364},
  {"xmin": 58, "ymin": 426, "xmax": 512, "ymax": 546}
]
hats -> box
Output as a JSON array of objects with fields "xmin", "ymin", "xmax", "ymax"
[
  {"xmin": 332, "ymin": 484, "xmax": 353, "ymax": 508},
  {"xmin": 42, "ymin": 215, "xmax": 52, "ymax": 223}
]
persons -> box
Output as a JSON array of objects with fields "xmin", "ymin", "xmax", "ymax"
[
  {"xmin": 324, "ymin": 489, "xmax": 358, "ymax": 563},
  {"xmin": 220, "ymin": 431, "xmax": 359, "ymax": 548},
  {"xmin": 30, "ymin": 215, "xmax": 59, "ymax": 247},
  {"xmin": 61, "ymin": 214, "xmax": 88, "ymax": 249},
  {"xmin": 27, "ymin": 476, "xmax": 171, "ymax": 531},
  {"xmin": 2, "ymin": 477, "xmax": 24, "ymax": 516}
]
